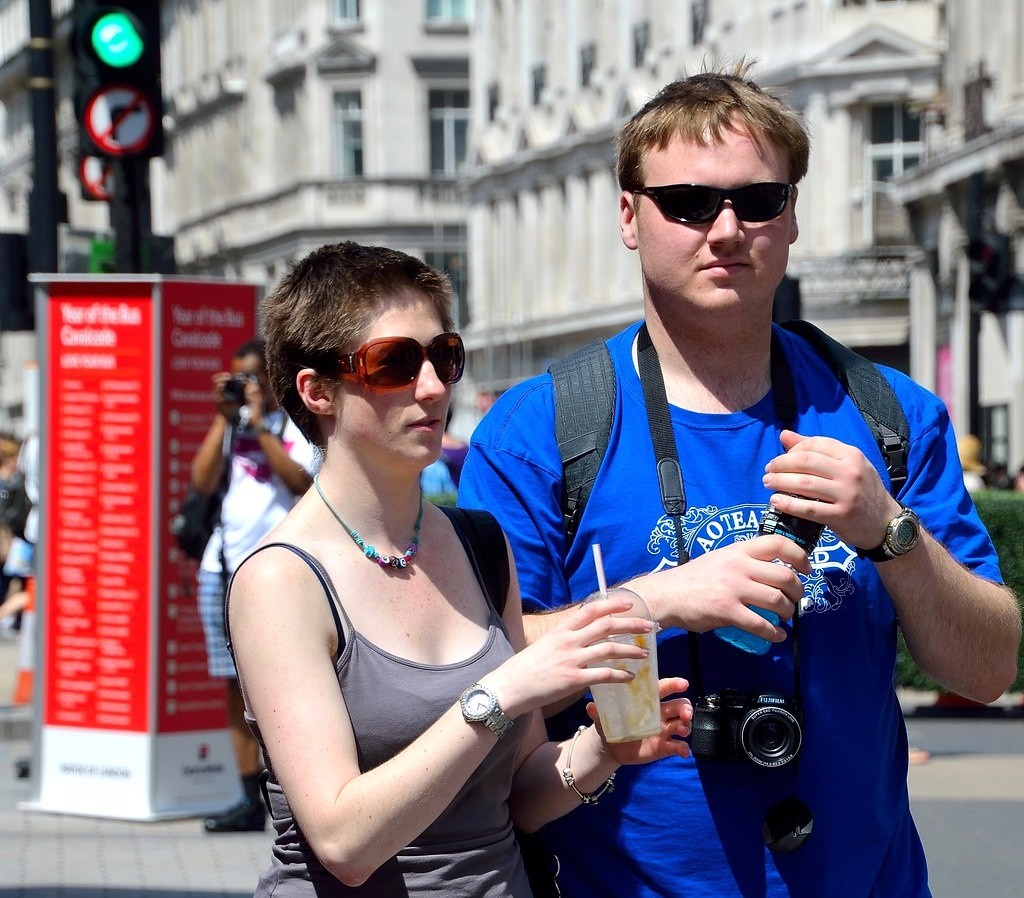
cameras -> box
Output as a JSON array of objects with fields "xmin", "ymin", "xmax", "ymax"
[
  {"xmin": 694, "ymin": 679, "xmax": 803, "ymax": 769},
  {"xmin": 223, "ymin": 372, "xmax": 259, "ymax": 405}
]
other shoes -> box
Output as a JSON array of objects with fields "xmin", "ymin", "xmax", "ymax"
[{"xmin": 206, "ymin": 802, "xmax": 267, "ymax": 832}]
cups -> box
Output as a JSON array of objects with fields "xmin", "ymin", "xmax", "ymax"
[
  {"xmin": 712, "ymin": 499, "xmax": 826, "ymax": 659},
  {"xmin": 580, "ymin": 587, "xmax": 665, "ymax": 749}
]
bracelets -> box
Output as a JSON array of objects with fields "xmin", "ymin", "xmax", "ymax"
[{"xmin": 563, "ymin": 725, "xmax": 616, "ymax": 805}]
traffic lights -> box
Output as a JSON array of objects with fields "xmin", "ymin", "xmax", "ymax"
[{"xmin": 961, "ymin": 232, "xmax": 1015, "ymax": 317}]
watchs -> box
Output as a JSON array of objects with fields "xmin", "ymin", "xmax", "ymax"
[
  {"xmin": 249, "ymin": 417, "xmax": 272, "ymax": 437},
  {"xmin": 854, "ymin": 499, "xmax": 922, "ymax": 563},
  {"xmin": 457, "ymin": 683, "xmax": 514, "ymax": 740}
]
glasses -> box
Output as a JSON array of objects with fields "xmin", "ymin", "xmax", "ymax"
[
  {"xmin": 286, "ymin": 332, "xmax": 467, "ymax": 388},
  {"xmin": 628, "ymin": 183, "xmax": 800, "ymax": 223}
]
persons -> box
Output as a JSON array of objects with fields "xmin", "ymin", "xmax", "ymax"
[
  {"xmin": 223, "ymin": 241, "xmax": 691, "ymax": 898},
  {"xmin": 185, "ymin": 338, "xmax": 326, "ymax": 833},
  {"xmin": 420, "ymin": 458, "xmax": 458, "ymax": 498},
  {"xmin": 457, "ymin": 55, "xmax": 1022, "ymax": 898},
  {"xmin": 470, "ymin": 391, "xmax": 493, "ymax": 431},
  {"xmin": 0, "ymin": 430, "xmax": 41, "ymax": 638},
  {"xmin": 956, "ymin": 433, "xmax": 988, "ymax": 494},
  {"xmin": 439, "ymin": 403, "xmax": 468, "ymax": 489},
  {"xmin": 992, "ymin": 463, "xmax": 1024, "ymax": 496}
]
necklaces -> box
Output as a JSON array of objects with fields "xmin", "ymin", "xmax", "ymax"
[{"xmin": 313, "ymin": 473, "xmax": 424, "ymax": 570}]
